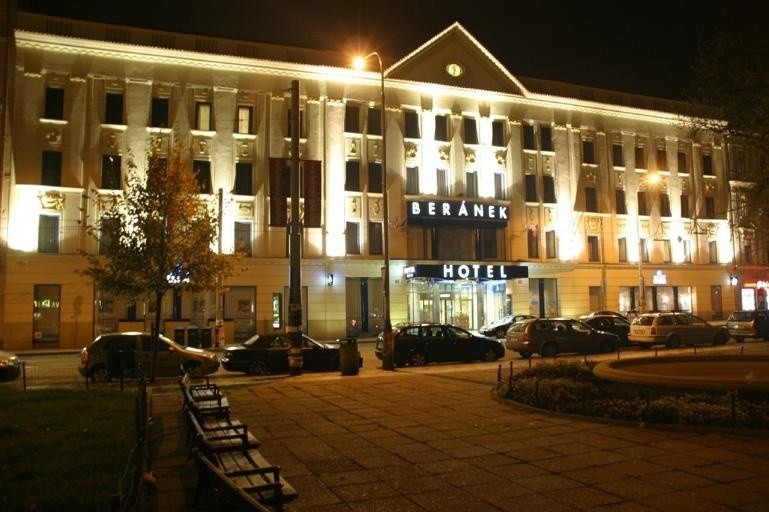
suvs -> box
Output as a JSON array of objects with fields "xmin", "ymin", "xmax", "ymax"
[
  {"xmin": 79, "ymin": 331, "xmax": 219, "ymax": 383},
  {"xmin": 727, "ymin": 309, "xmax": 769, "ymax": 342}
]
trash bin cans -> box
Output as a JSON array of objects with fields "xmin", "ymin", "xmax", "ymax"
[{"xmin": 340, "ymin": 338, "xmax": 359, "ymax": 375}]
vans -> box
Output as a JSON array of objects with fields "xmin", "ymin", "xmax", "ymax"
[
  {"xmin": 375, "ymin": 323, "xmax": 505, "ymax": 368},
  {"xmin": 506, "ymin": 316, "xmax": 621, "ymax": 358}
]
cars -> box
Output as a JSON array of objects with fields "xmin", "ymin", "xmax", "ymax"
[
  {"xmin": 582, "ymin": 316, "xmax": 638, "ymax": 346},
  {"xmin": 479, "ymin": 314, "xmax": 538, "ymax": 339},
  {"xmin": 223, "ymin": 331, "xmax": 363, "ymax": 376},
  {"xmin": 579, "ymin": 310, "xmax": 628, "ymax": 322},
  {"xmin": 626, "ymin": 312, "xmax": 730, "ymax": 349},
  {"xmin": 0, "ymin": 349, "xmax": 21, "ymax": 381}
]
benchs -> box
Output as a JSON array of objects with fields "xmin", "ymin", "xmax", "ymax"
[{"xmin": 174, "ymin": 370, "xmax": 301, "ymax": 511}]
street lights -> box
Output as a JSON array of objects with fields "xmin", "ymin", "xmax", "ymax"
[
  {"xmin": 352, "ymin": 51, "xmax": 395, "ymax": 371},
  {"xmin": 636, "ymin": 175, "xmax": 662, "ymax": 315}
]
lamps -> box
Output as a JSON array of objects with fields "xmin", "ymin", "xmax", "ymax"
[
  {"xmin": 730, "ymin": 275, "xmax": 739, "ymax": 286},
  {"xmin": 327, "ymin": 273, "xmax": 334, "ymax": 288}
]
